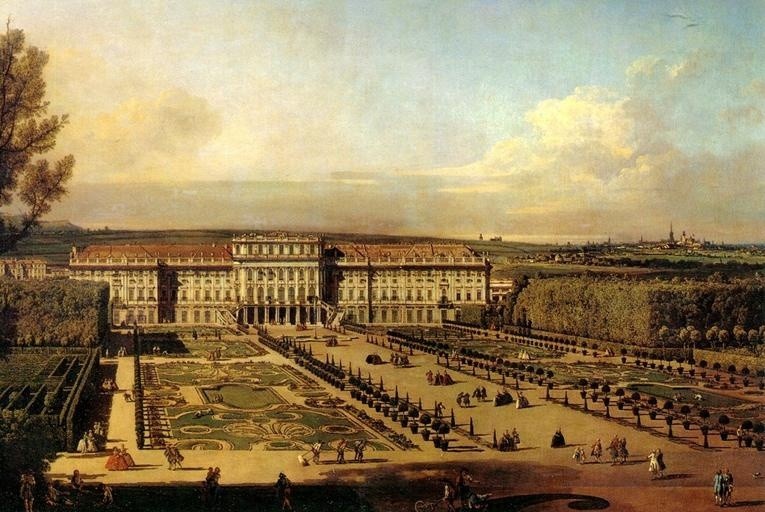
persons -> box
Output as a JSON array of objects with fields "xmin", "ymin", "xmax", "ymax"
[
  {"xmin": 387, "ymin": 351, "xmax": 703, "ymax": 511},
  {"xmin": 736, "ymin": 424, "xmax": 745, "ymax": 448},
  {"xmin": 100, "ymin": 377, "xmax": 136, "ymax": 402},
  {"xmin": 17, "ymin": 420, "xmax": 221, "ymax": 512},
  {"xmin": 117, "ymin": 343, "xmax": 222, "ymax": 361},
  {"xmin": 713, "ymin": 470, "xmax": 725, "ymax": 505},
  {"xmin": 311, "ymin": 437, "xmax": 367, "ymax": 465},
  {"xmin": 270, "ymin": 471, "xmax": 293, "ymax": 511},
  {"xmin": 722, "ymin": 468, "xmax": 734, "ymax": 506}
]
